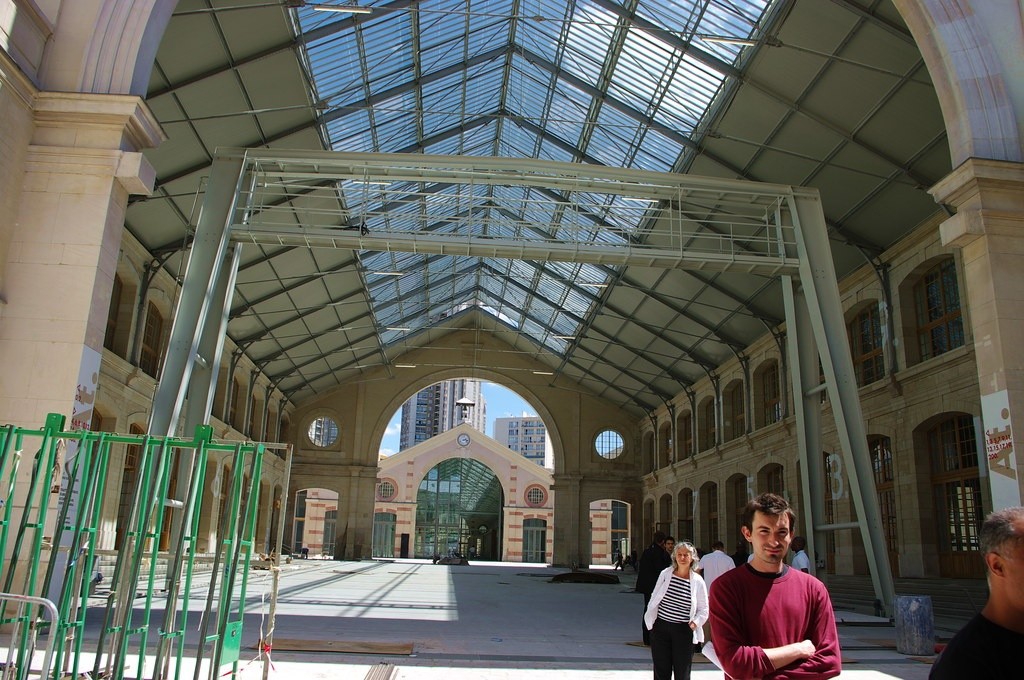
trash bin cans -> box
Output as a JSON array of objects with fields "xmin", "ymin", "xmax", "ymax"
[{"xmin": 892, "ymin": 594, "xmax": 936, "ymax": 656}]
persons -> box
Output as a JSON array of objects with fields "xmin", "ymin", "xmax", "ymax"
[
  {"xmin": 644, "ymin": 541, "xmax": 711, "ymax": 680},
  {"xmin": 707, "ymin": 492, "xmax": 844, "ymax": 680},
  {"xmin": 927, "ymin": 508, "xmax": 1024, "ymax": 680},
  {"xmin": 469, "ymin": 545, "xmax": 476, "ymax": 561},
  {"xmin": 613, "ymin": 549, "xmax": 624, "ymax": 571},
  {"xmin": 634, "ymin": 532, "xmax": 812, "ymax": 644}
]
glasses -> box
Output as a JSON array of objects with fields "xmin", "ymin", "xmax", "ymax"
[{"xmin": 666, "ymin": 542, "xmax": 674, "ymax": 545}]
